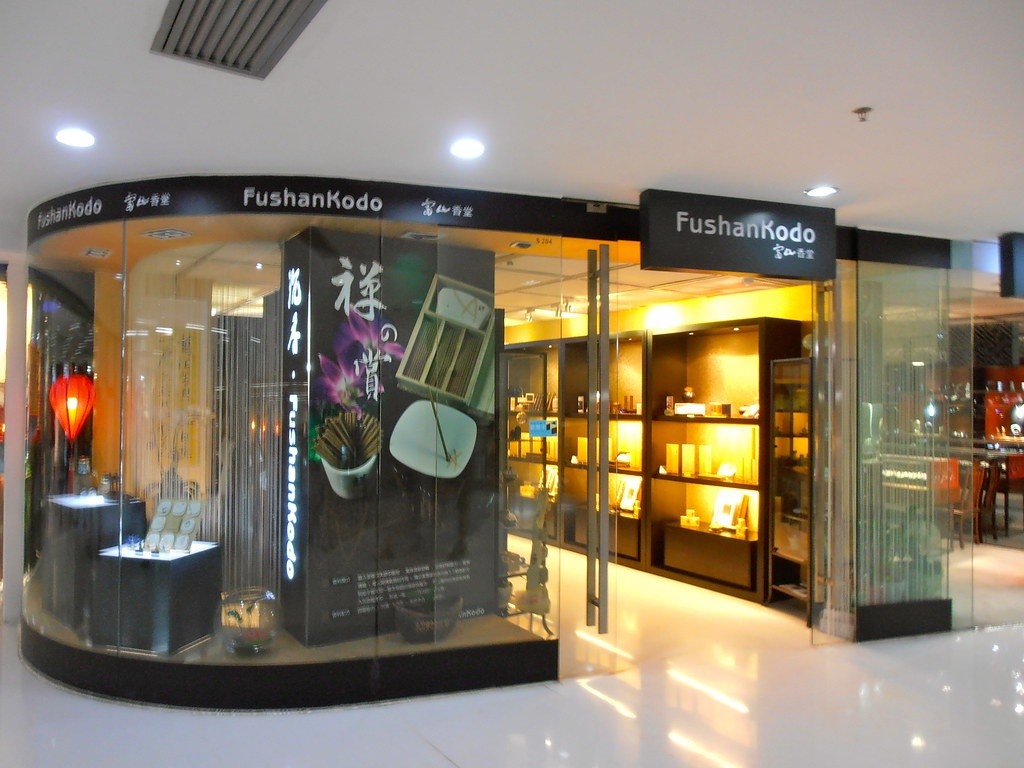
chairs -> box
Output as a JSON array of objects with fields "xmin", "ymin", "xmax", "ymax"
[{"xmin": 941, "ymin": 453, "xmax": 1024, "ymax": 548}]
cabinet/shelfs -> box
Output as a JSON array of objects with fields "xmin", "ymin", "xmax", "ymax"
[{"xmin": 504, "ymin": 317, "xmax": 813, "ymax": 629}]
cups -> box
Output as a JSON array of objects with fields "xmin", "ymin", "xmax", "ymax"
[{"xmin": 321, "ymin": 454, "xmax": 376, "ymax": 499}]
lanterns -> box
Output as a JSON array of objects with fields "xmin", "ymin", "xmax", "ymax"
[{"xmin": 49, "ymin": 375, "xmax": 96, "ymax": 445}]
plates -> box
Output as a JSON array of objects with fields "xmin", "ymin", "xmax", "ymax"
[{"xmin": 390, "ymin": 401, "xmax": 477, "ymax": 478}]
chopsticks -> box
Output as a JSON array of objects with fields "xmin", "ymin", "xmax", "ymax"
[{"xmin": 313, "ymin": 411, "xmax": 382, "ymax": 469}]
